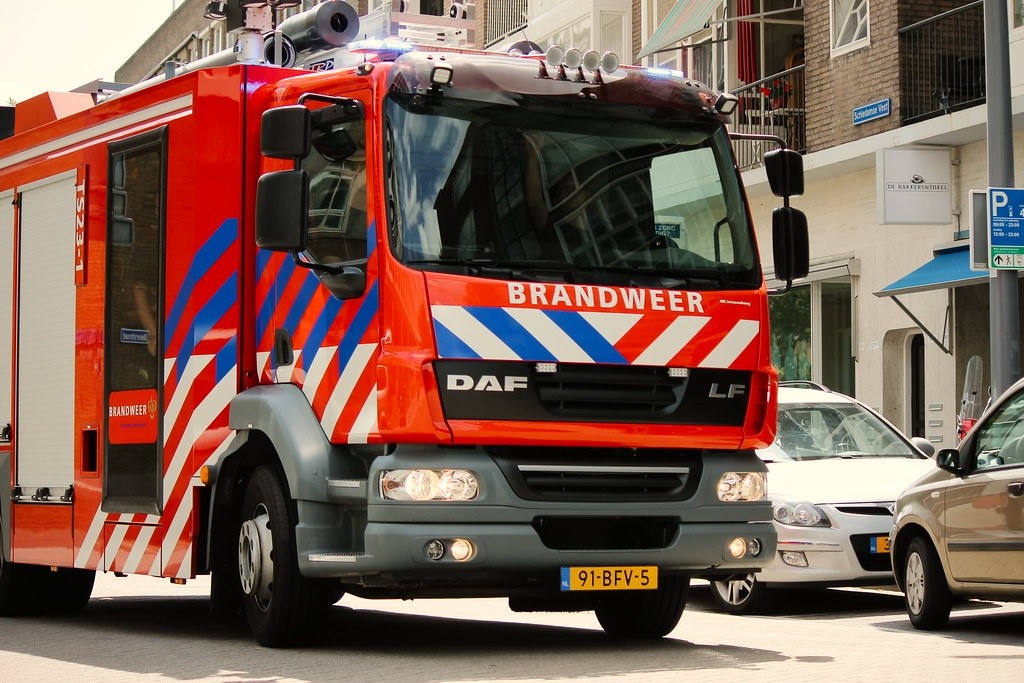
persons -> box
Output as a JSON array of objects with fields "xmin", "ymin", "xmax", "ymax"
[
  {"xmin": 538, "ymin": 172, "xmax": 625, "ymax": 252},
  {"xmin": 134, "ymin": 250, "xmax": 164, "ymax": 389}
]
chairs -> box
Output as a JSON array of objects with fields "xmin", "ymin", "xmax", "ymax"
[
  {"xmin": 745, "ymin": 110, "xmax": 783, "ymax": 170},
  {"xmin": 779, "ymin": 419, "xmax": 823, "ymax": 458}
]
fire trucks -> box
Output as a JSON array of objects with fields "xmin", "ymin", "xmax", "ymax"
[{"xmin": 6, "ymin": 2, "xmax": 812, "ymax": 653}]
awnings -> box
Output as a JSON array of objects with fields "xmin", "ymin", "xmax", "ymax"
[
  {"xmin": 872, "ymin": 246, "xmax": 1024, "ymax": 298},
  {"xmin": 634, "ymin": 0, "xmax": 724, "ymax": 60}
]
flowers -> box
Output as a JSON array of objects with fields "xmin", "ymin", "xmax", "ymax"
[{"xmin": 760, "ymin": 78, "xmax": 793, "ymax": 99}]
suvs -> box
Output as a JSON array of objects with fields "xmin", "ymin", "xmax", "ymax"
[{"xmin": 709, "ymin": 379, "xmax": 938, "ymax": 615}]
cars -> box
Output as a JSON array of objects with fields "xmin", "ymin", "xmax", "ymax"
[{"xmin": 887, "ymin": 377, "xmax": 1024, "ymax": 632}]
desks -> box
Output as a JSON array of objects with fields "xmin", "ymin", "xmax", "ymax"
[{"xmin": 763, "ymin": 108, "xmax": 807, "ymax": 150}]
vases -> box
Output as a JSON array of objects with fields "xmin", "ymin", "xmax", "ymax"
[{"xmin": 769, "ymin": 96, "xmax": 789, "ymax": 110}]
